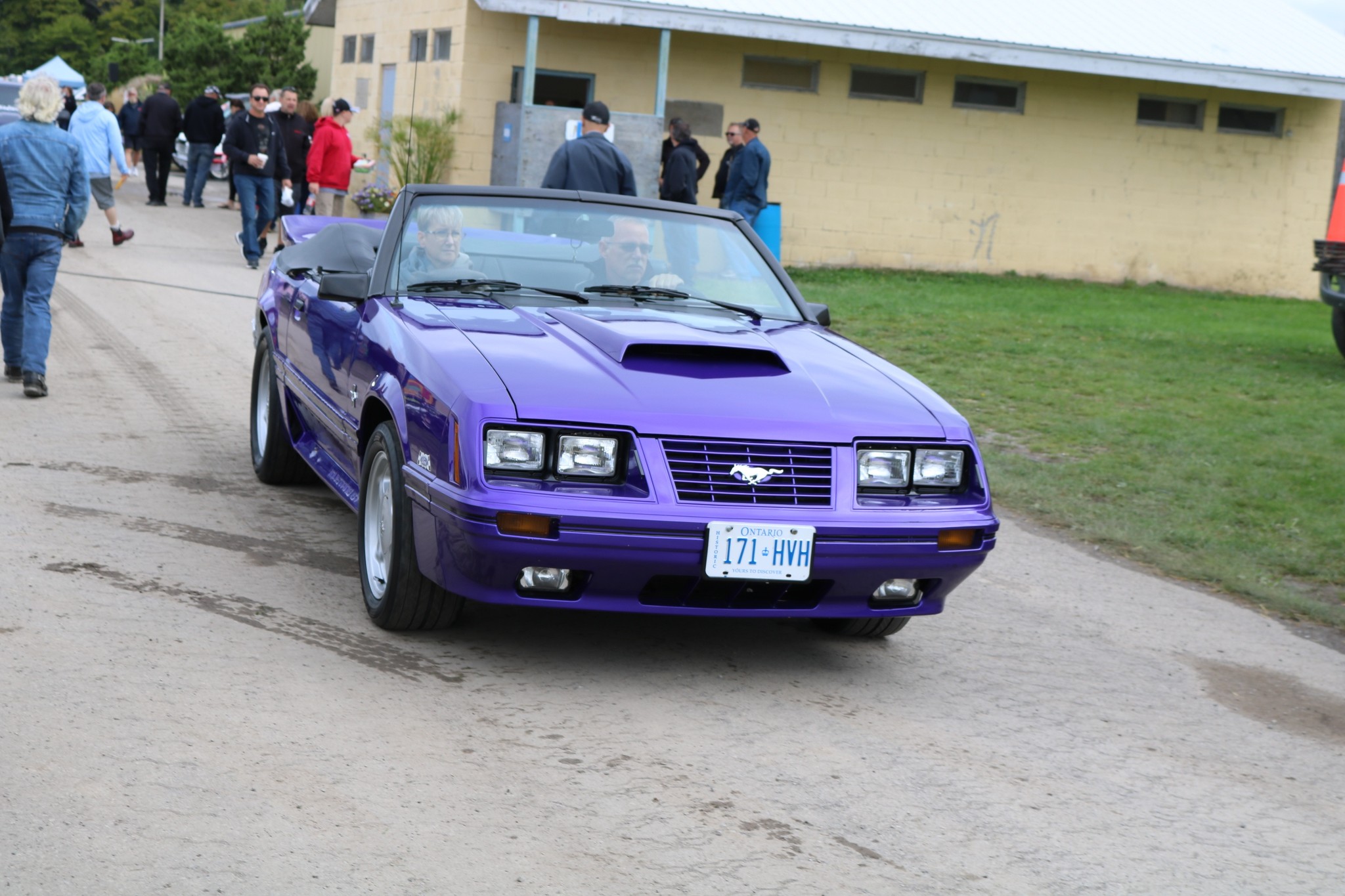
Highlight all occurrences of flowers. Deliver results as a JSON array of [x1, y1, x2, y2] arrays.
[[351, 185, 397, 214]]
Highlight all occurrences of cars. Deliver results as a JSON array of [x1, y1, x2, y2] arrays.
[[173, 90, 252, 179], [0, 73, 27, 125]]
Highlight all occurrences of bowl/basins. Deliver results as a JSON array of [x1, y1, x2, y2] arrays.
[[353, 159, 370, 173]]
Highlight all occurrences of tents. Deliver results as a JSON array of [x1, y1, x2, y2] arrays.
[[22, 54, 84, 89]]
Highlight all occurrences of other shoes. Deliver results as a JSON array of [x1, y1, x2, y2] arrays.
[[245, 260, 259, 269], [22, 370, 48, 398], [259, 237, 267, 257], [194, 203, 204, 208], [69, 239, 84, 247], [273, 244, 285, 254], [3, 365, 23, 383], [235, 232, 248, 260], [111, 228, 134, 245], [146, 199, 167, 206], [182, 200, 190, 206]]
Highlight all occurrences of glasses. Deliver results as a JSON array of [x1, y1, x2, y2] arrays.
[[282, 86, 296, 92], [251, 95, 268, 102], [724, 130, 740, 136], [604, 239, 653, 254], [425, 229, 466, 242]]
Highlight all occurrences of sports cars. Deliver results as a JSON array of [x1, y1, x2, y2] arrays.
[[247, 181, 1002, 642]]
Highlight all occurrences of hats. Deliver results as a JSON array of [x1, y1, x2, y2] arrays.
[[739, 118, 760, 132], [205, 85, 224, 99], [333, 98, 360, 114], [583, 100, 610, 125]]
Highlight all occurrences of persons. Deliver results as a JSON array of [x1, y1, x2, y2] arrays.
[[660, 117, 770, 277], [390, 204, 488, 290], [573, 215, 685, 290], [0, 73, 366, 397], [522, 100, 637, 246]]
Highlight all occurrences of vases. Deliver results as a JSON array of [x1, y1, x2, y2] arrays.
[[359, 212, 395, 220]]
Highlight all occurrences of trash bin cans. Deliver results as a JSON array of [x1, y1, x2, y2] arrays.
[[754, 200, 782, 261]]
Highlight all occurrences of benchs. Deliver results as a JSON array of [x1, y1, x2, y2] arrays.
[[407, 227, 598, 287]]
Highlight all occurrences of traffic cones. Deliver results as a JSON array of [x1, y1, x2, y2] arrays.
[[1310, 158, 1345, 273]]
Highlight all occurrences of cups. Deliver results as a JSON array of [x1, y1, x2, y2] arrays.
[[257, 153, 268, 169]]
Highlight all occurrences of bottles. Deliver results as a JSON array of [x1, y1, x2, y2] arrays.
[[303, 192, 316, 215]]
